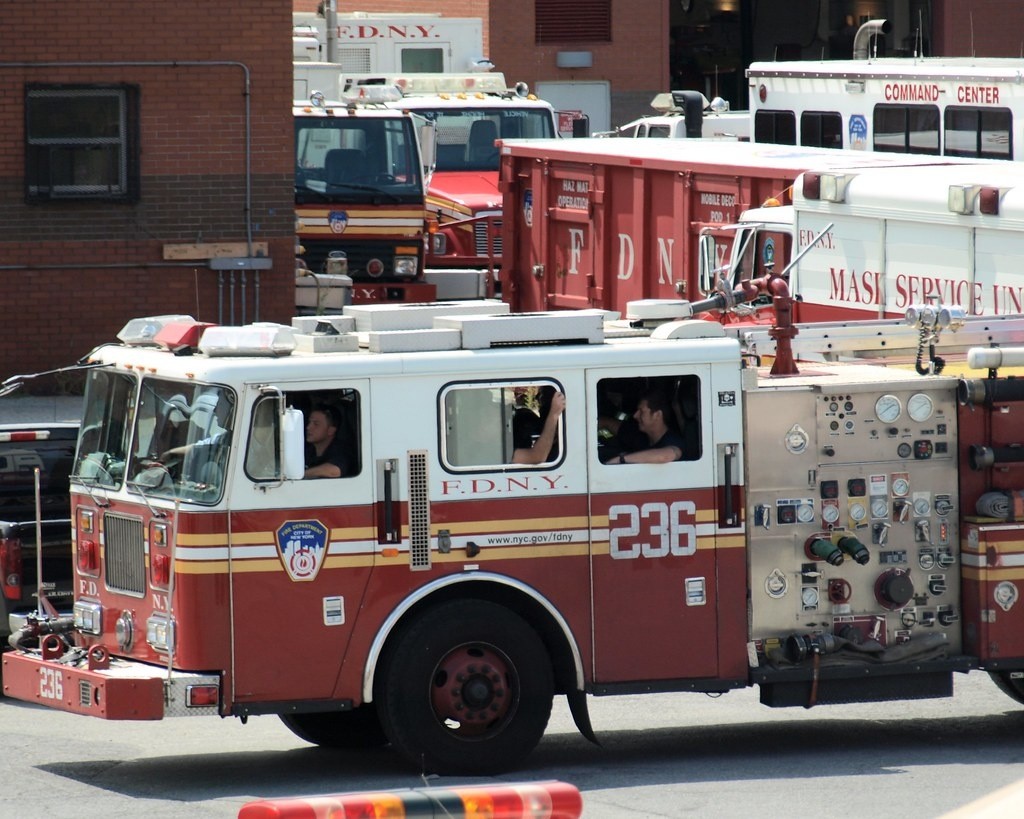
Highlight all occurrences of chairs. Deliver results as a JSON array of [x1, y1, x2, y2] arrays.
[[467, 121, 501, 162], [398, 141, 445, 167], [335, 398, 359, 478], [325, 149, 372, 182]]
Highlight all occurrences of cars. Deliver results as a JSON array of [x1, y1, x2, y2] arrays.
[[0, 367, 93, 610]]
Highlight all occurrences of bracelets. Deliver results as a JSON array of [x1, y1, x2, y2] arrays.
[[619, 452, 625, 463]]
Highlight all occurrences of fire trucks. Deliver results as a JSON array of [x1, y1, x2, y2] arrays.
[[2, 161, 1024, 774], [488, 16, 1023, 337], [291, 0, 595, 307]]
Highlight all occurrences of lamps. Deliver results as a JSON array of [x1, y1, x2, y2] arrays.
[[555, 51, 593, 68]]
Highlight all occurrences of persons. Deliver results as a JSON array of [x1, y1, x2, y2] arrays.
[[304, 406, 346, 478], [512, 387, 566, 465], [161, 389, 233, 488], [597, 394, 687, 465]]
